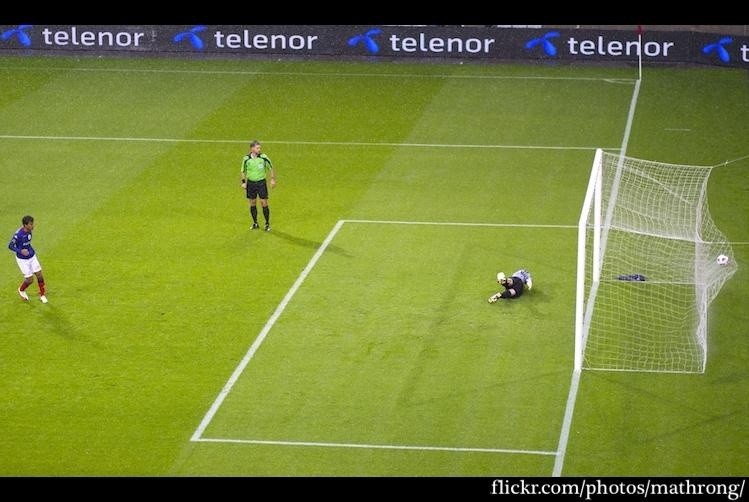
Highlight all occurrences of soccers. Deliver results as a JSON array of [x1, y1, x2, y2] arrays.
[[717, 255, 728, 265]]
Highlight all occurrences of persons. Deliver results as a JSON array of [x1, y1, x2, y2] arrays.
[[487, 268, 533, 304], [241, 140, 276, 231], [8, 215, 49, 304]]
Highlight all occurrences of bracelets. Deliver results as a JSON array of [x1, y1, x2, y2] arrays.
[[242, 179, 245, 184]]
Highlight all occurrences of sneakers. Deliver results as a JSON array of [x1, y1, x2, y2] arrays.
[[18, 289, 29, 301], [250, 223, 270, 231], [40, 295, 48, 303]]
